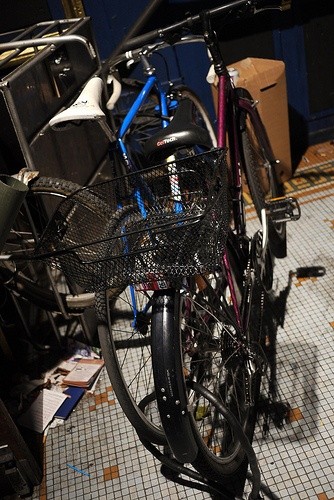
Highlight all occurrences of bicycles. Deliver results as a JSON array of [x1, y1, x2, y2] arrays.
[[0, 1, 326, 493]]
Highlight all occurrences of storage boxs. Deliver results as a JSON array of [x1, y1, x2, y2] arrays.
[[212, 57, 292, 197]]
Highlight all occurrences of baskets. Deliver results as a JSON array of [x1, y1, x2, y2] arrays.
[[12, 145, 231, 292]]
